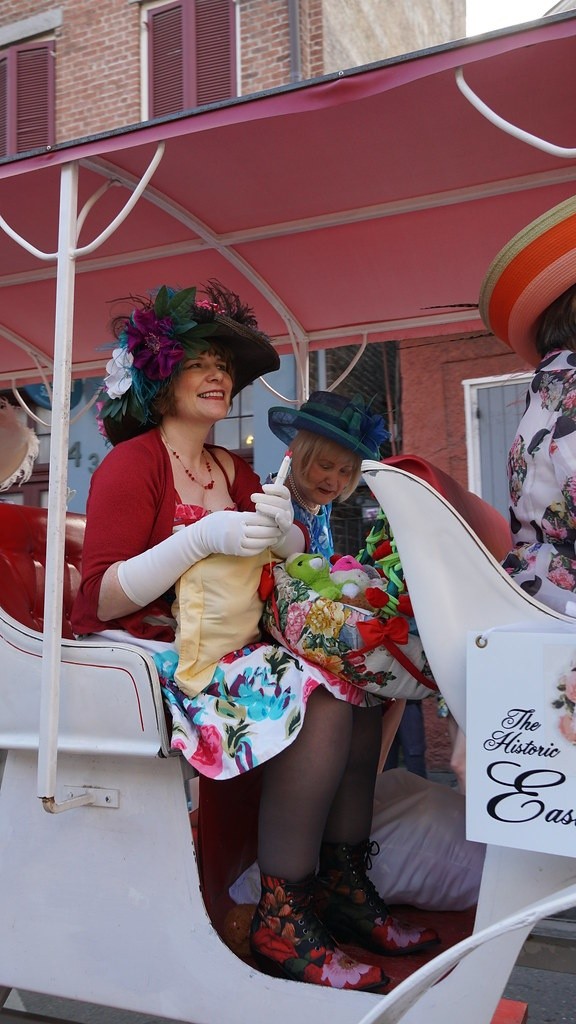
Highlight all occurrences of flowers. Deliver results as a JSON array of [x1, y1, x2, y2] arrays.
[[93, 283, 226, 446]]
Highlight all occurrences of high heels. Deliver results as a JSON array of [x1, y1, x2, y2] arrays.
[[251, 870, 391, 990], [319, 839, 441, 955]]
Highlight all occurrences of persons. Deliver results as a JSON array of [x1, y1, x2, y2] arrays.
[[510, 262, 576, 621], [70, 328, 442, 990], [265, 418, 407, 778]]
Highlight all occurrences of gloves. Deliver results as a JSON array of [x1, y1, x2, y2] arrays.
[[117, 511, 282, 607], [250, 483, 306, 560]]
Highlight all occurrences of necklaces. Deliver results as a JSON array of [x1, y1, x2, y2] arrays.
[[162, 437, 215, 490]]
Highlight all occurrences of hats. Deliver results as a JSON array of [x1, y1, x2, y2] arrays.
[[1, 400, 38, 490], [103, 306, 280, 446], [267, 390, 381, 460], [480, 196, 575, 368]]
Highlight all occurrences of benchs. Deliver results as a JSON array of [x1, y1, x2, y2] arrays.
[[0, 501, 199, 781], [362, 456, 575, 738]]
